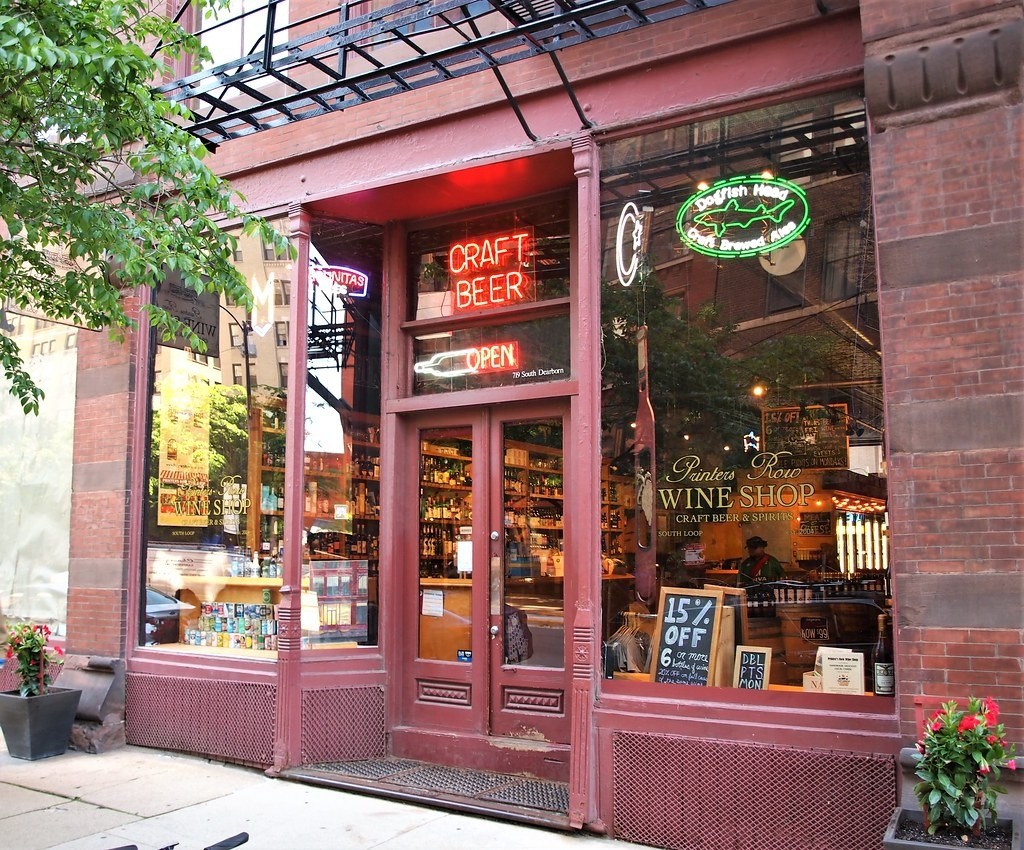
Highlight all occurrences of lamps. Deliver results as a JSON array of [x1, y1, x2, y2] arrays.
[[756, 225, 806, 279]]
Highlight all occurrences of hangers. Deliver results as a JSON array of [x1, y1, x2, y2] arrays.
[[606, 612, 645, 647]]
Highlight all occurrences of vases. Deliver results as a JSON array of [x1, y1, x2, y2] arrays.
[[0, 686, 83, 762], [882, 807, 1021, 850]]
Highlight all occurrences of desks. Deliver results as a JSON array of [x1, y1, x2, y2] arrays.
[[505, 574, 637, 642]]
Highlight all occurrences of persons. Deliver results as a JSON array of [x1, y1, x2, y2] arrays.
[[740, 536, 785, 582]]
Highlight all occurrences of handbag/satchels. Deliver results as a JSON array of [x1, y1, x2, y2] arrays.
[[504, 610, 534, 663]]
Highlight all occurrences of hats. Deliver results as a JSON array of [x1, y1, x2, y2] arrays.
[[745, 536, 767, 549]]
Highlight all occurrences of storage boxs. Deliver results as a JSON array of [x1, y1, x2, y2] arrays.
[[546, 555, 564, 577], [602, 558, 627, 575], [373, 465, 380, 477]]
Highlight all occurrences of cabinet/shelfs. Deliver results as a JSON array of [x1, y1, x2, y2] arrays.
[[245, 389, 639, 580], [178, 574, 358, 661]]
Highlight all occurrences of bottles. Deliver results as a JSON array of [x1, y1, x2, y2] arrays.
[[600, 483, 621, 556], [872, 614, 895, 697], [420, 456, 473, 578], [747, 560, 892, 608], [230, 408, 287, 579], [304, 426, 379, 577], [504, 451, 564, 577]]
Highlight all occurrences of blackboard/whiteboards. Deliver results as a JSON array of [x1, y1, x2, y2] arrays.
[[650, 586, 724, 686], [732, 645, 771, 690], [703, 584, 748, 660]]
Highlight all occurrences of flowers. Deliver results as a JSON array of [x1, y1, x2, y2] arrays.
[[3, 620, 66, 698], [910, 694, 1018, 838]]
